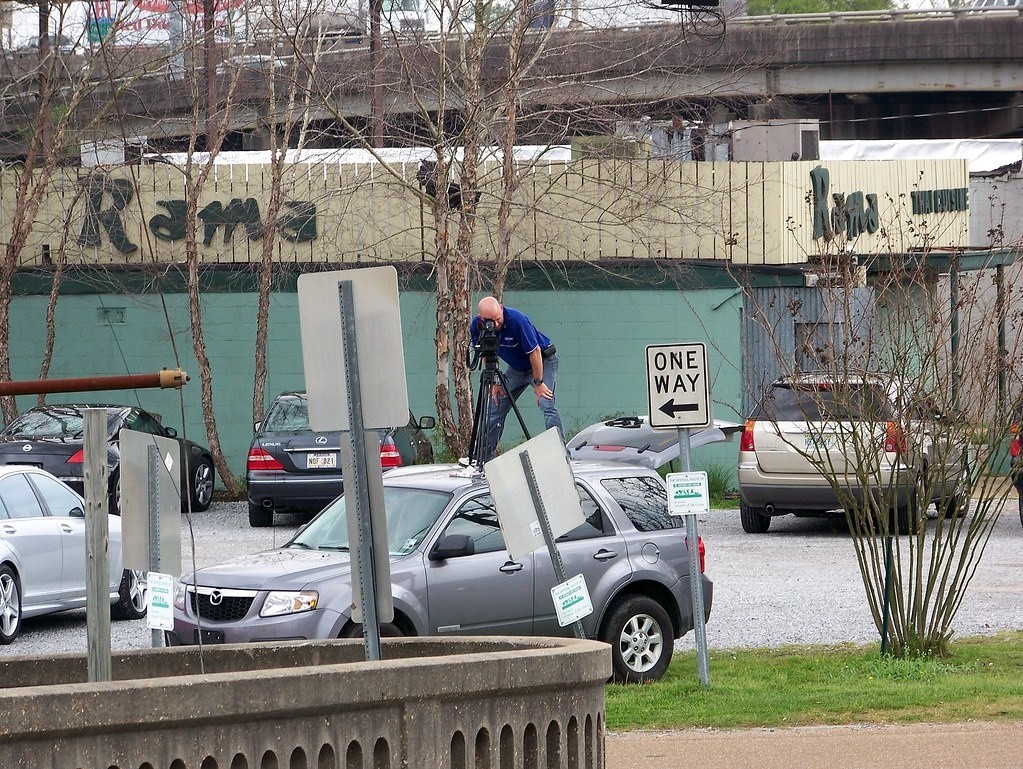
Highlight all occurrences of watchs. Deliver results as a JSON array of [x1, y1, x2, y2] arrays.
[[534, 376, 544, 385]]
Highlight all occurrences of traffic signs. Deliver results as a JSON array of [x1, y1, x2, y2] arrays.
[[645, 341, 713, 432]]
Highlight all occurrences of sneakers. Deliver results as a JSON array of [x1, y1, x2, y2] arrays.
[[458, 456, 484, 466]]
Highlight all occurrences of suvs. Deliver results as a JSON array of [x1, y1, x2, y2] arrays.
[[735, 371, 973, 537], [163, 413, 746, 684], [246, 391, 436, 527]]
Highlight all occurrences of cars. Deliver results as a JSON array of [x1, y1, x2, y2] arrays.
[[1008, 386, 1023, 528], [0, 462, 157, 646], [0, 404, 217, 517]]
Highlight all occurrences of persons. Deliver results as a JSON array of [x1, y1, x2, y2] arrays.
[[456, 296, 572, 462]]
